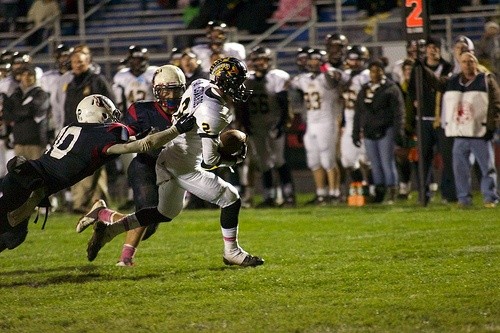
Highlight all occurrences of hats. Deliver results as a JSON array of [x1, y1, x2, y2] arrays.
[[120, 44, 150, 65], [403, 37, 441, 66], [454, 35, 478, 63], [368, 59, 385, 69], [70, 44, 90, 55]]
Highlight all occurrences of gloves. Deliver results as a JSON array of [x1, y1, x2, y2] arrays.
[[395, 134, 408, 147], [221, 143, 248, 167], [175, 113, 196, 134], [352, 132, 360, 147]]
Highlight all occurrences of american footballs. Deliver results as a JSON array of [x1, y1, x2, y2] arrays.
[[218, 129, 246, 152]]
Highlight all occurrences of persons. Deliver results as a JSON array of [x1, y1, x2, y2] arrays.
[[190, 22, 246, 78], [479, 0, 500, 21], [341, 0, 403, 17], [178, 0, 317, 40], [86, 57, 265, 268], [426, 0, 474, 21], [0, 44, 210, 216], [0, 0, 100, 52], [0, 94, 195, 254], [74, 64, 188, 266], [237, 19, 500, 209]]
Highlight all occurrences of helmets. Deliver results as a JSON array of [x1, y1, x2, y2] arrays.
[[170, 48, 182, 58], [152, 65, 187, 103], [75, 94, 122, 124], [206, 20, 230, 45], [56, 44, 70, 56], [210, 57, 254, 104], [295, 33, 371, 60], [0, 51, 30, 72], [250, 46, 273, 72]]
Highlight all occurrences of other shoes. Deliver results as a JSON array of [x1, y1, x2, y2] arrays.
[[255, 197, 296, 208], [222, 248, 264, 267], [374, 190, 384, 203], [115, 260, 137, 267], [76, 199, 107, 233], [304, 194, 343, 207], [86, 220, 109, 261], [484, 200, 495, 208]]
